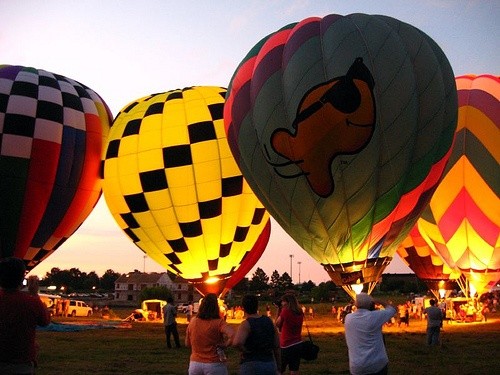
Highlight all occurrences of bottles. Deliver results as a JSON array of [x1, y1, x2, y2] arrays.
[[217, 348, 227, 361]]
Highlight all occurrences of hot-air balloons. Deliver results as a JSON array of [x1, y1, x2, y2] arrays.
[[100, 85, 271, 317], [223, 13, 459, 304], [395, 223, 459, 318], [415, 73, 499, 323], [183, 219, 271, 309], [0, 65, 114, 290]]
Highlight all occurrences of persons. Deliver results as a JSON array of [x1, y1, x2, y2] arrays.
[[0, 257, 53, 375], [185, 293, 233, 375], [229, 295, 285, 375], [297, 303, 345, 321], [52, 296, 70, 318], [419, 298, 444, 349], [92, 304, 114, 320], [271, 292, 305, 375], [162, 298, 184, 350], [344, 294, 397, 375], [129, 300, 272, 318], [373, 282, 500, 323]]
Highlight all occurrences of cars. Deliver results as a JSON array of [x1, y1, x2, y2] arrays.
[[177, 304, 190, 313], [339, 305, 352, 323]]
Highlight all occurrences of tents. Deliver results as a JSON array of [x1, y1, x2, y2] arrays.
[[141, 299, 167, 323]]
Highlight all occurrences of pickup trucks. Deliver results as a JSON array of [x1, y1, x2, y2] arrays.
[[68, 300, 93, 318]]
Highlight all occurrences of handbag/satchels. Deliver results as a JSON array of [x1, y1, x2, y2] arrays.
[[301, 341, 319, 361]]
